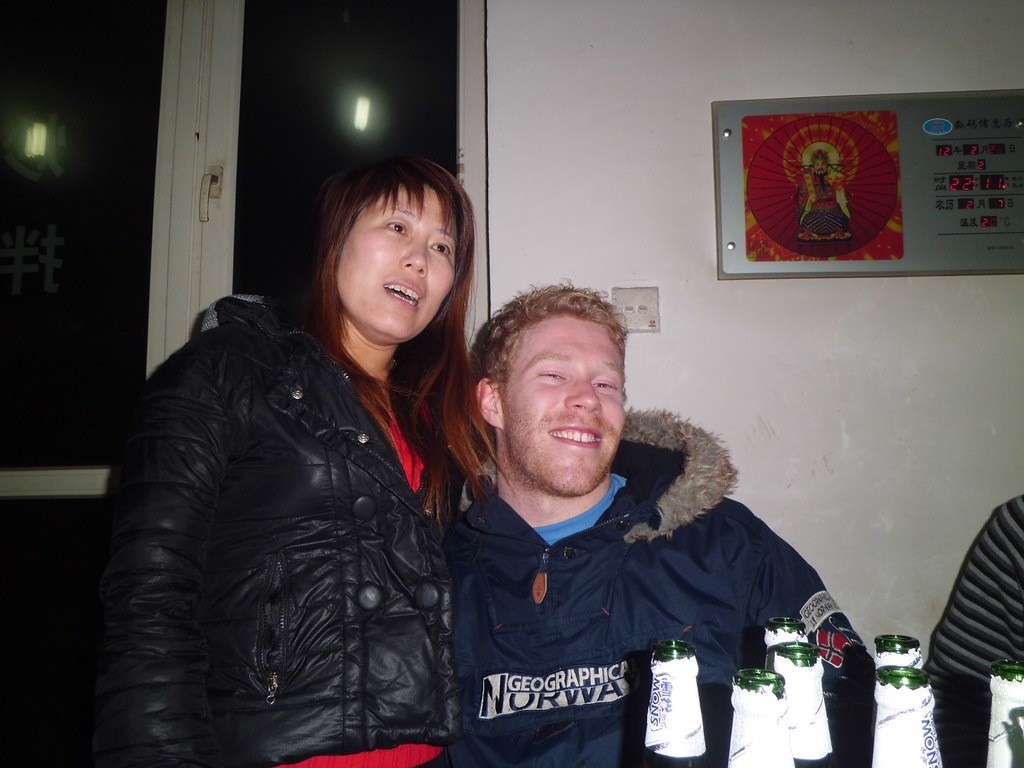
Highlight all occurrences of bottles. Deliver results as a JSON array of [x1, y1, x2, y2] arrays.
[[639, 616, 1024, 768]]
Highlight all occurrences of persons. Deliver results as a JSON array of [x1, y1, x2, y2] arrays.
[[94, 155, 503, 768], [923, 493, 1024, 768], [439, 287, 876, 768]]
